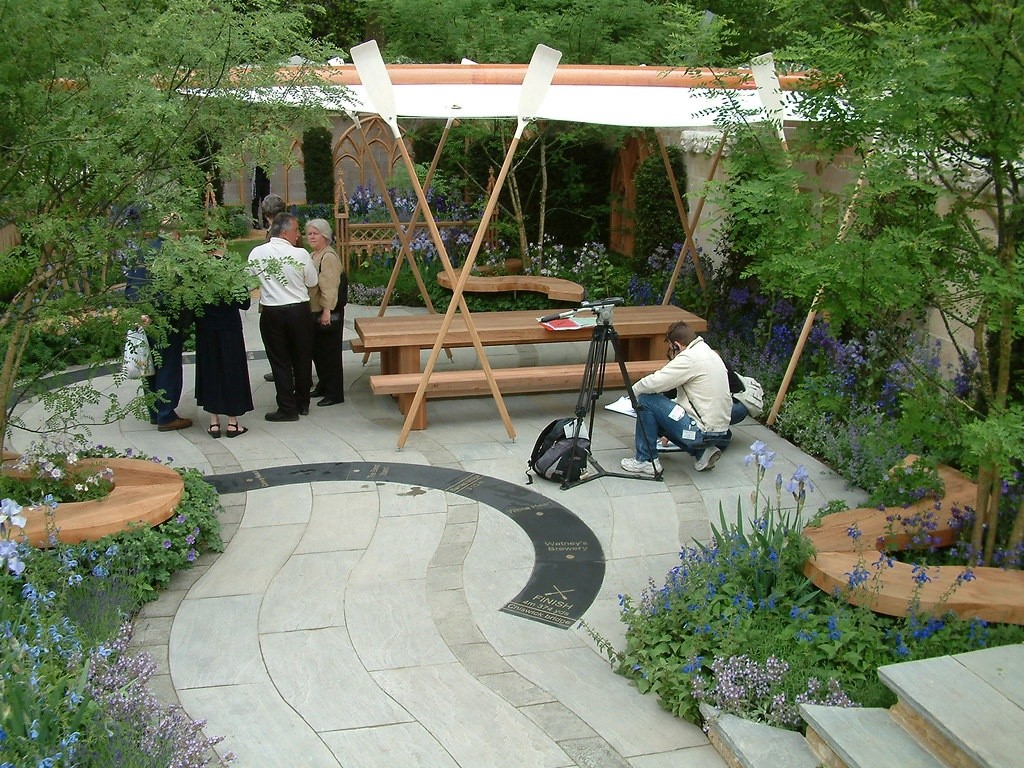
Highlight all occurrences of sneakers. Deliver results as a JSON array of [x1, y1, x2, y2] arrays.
[[693, 446, 722, 472], [621, 457, 664, 475]]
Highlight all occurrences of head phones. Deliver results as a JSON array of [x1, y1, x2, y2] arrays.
[[667, 334, 680, 350]]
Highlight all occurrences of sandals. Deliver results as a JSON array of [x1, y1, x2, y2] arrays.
[[207, 423, 221, 438], [226, 422, 248, 438]]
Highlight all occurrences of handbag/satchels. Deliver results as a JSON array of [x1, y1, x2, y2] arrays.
[[123, 325, 156, 379], [732, 377, 764, 418], [318, 251, 348, 313]]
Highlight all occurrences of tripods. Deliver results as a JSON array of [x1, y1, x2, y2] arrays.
[[541, 305, 664, 482]]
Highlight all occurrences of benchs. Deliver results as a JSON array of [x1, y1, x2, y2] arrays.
[[370, 358, 687, 431], [437, 259, 584, 302], [350, 338, 381, 366], [0, 452, 185, 550], [799, 453, 1024, 625]]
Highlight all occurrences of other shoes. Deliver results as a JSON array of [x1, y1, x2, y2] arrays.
[[293, 376, 313, 390], [317, 397, 344, 406], [150, 418, 158, 424], [310, 389, 322, 397], [265, 410, 299, 421], [300, 408, 309, 415], [264, 373, 275, 381], [158, 417, 193, 431]]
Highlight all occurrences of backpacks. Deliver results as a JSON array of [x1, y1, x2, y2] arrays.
[[525, 418, 592, 485]]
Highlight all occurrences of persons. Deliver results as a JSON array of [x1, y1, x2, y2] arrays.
[[656, 344, 765, 447], [258, 194, 313, 383], [244, 212, 319, 422], [125, 212, 255, 439], [621, 319, 733, 475], [305, 218, 349, 406]]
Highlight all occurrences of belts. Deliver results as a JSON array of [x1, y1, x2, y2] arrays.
[[264, 301, 310, 310]]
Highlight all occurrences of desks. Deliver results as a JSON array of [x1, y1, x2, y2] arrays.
[[354, 305, 707, 414]]
